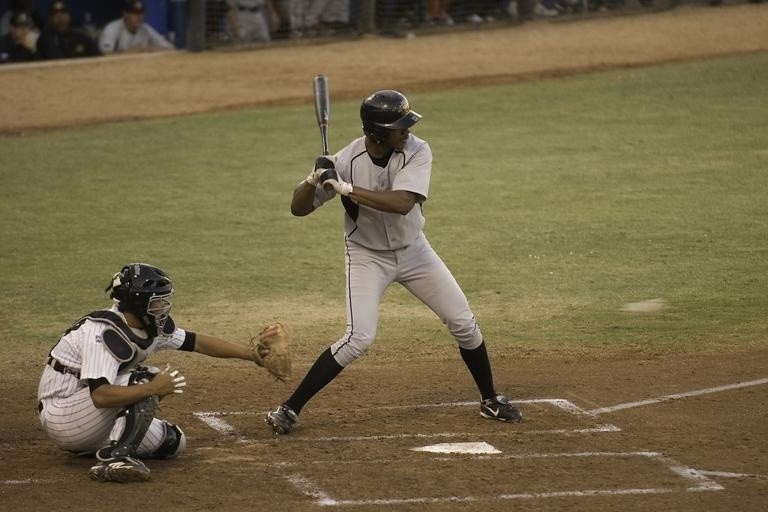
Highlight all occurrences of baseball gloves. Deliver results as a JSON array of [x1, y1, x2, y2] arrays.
[[256, 325, 292, 377]]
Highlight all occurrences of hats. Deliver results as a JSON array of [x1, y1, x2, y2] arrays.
[[9, 0, 144, 28]]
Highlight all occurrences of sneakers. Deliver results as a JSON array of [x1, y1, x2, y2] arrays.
[[88, 458, 153, 484], [480, 395, 522, 422], [263, 402, 298, 434]]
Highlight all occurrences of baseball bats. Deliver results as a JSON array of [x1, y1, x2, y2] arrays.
[[312, 76, 335, 191]]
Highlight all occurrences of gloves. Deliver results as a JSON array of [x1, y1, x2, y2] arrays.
[[304, 154, 354, 198]]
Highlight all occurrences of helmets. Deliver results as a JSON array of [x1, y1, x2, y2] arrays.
[[103, 262, 177, 338], [357, 88, 423, 131]]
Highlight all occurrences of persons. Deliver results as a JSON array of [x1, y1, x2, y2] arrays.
[[36, 262, 293, 484], [265, 89, 524, 436], [213, 0, 587, 42], [1, 0, 173, 65]]
